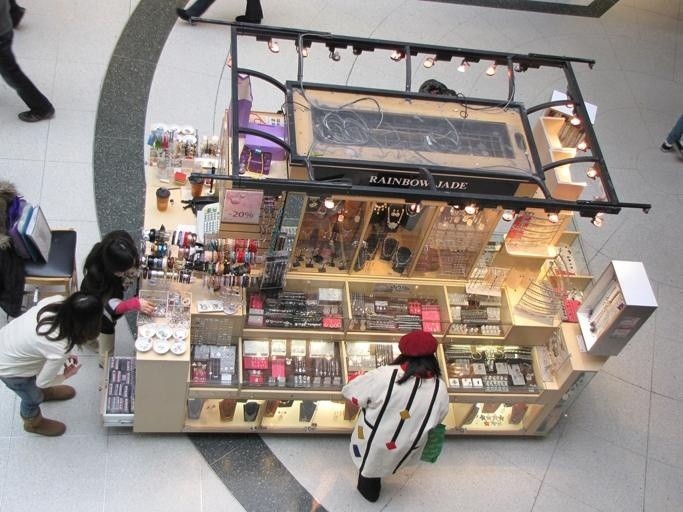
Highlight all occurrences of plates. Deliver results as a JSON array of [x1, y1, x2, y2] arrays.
[[134, 323, 188, 355]]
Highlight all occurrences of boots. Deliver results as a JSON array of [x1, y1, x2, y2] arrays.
[[21, 408, 65, 436], [40, 385, 75, 401]]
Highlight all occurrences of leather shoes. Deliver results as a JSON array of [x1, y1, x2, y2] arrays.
[[19, 109, 55, 121]]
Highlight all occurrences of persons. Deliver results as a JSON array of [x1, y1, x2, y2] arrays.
[[340, 331, 449, 503], [175, 0, 263, 24], [0, 1, 55, 123], [660, 112, 683, 165], [0, 291, 101, 436], [79, 229, 156, 371]]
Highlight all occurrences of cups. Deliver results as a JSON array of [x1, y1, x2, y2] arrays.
[[155, 188, 170, 212], [189, 175, 204, 197]]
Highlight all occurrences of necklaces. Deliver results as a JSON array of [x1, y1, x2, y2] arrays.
[[190, 289, 344, 389], [345, 291, 539, 388], [293, 197, 490, 281]]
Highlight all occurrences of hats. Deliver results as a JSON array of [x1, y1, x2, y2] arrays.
[[398, 330, 437, 357]]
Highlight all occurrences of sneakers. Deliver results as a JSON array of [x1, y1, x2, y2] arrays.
[[661, 142, 674, 152], [86, 338, 99, 353], [236, 15, 261, 23], [175, 7, 196, 22]]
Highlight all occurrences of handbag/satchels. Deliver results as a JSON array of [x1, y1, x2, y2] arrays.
[[8, 196, 31, 258], [421, 424, 445, 462]]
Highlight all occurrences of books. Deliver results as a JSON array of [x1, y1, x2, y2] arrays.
[[10, 203, 52, 262]]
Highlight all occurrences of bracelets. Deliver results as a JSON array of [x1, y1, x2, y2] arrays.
[[141, 225, 266, 290]]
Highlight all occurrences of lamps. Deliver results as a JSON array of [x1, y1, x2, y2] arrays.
[[566, 83, 583, 128], [253, 36, 528, 75]]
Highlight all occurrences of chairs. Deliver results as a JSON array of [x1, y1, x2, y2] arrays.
[[16, 230, 77, 298]]
